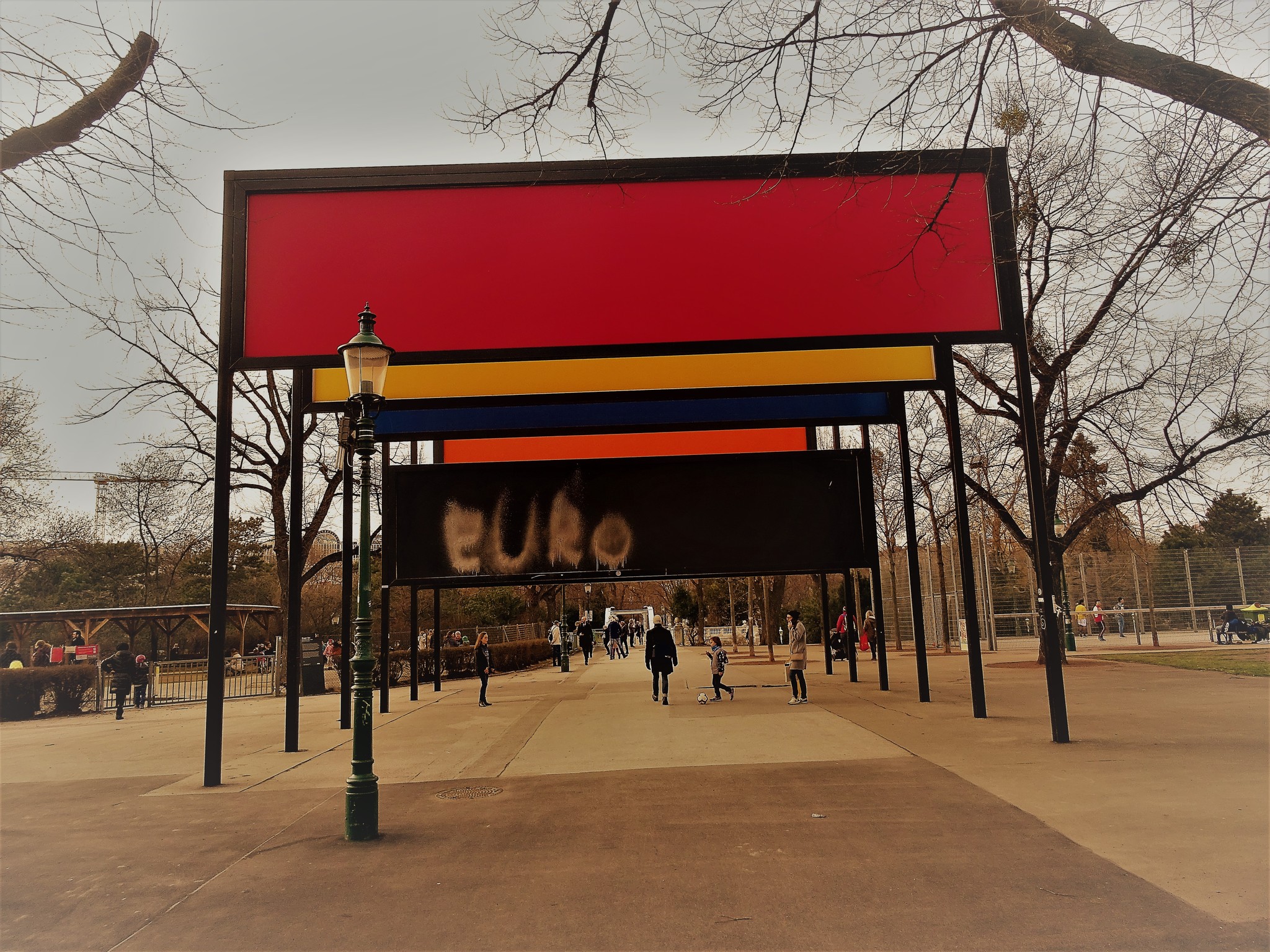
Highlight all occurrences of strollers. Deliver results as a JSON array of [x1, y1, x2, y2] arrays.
[[830, 628, 858, 662]]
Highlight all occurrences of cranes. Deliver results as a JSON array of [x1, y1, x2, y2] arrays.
[[0, 470, 173, 545]]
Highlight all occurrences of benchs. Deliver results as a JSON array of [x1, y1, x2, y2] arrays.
[[1218, 631, 1257, 645]]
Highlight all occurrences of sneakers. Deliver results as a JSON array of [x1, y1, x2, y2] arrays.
[[799, 696, 808, 703], [788, 695, 800, 705]]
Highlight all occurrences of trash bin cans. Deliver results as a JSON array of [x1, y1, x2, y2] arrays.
[[1240, 602, 1269, 641]]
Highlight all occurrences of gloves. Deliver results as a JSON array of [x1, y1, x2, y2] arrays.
[[673, 661, 678, 667], [646, 663, 651, 670]]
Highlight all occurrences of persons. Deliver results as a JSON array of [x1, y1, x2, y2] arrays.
[[576, 617, 594, 665], [837, 607, 857, 662], [474, 632, 495, 707], [101, 642, 149, 720], [1074, 599, 1088, 638], [863, 610, 877, 661], [1215, 604, 1238, 645], [170, 643, 179, 661], [1113, 597, 1127, 638], [1092, 600, 1108, 641], [322, 639, 341, 670], [225, 641, 273, 676], [547, 620, 562, 667], [645, 615, 678, 705], [0, 630, 85, 669], [706, 636, 735, 701], [786, 610, 808, 705], [602, 616, 646, 660], [417, 628, 470, 650]]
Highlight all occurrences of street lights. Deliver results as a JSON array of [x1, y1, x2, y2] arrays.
[[583, 583, 592, 625], [661, 604, 665, 626], [336, 300, 397, 842]]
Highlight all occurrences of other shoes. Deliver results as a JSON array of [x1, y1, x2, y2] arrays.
[[134, 704, 140, 708], [482, 697, 492, 706], [622, 652, 628, 658], [478, 698, 486, 707], [140, 704, 144, 709], [617, 653, 621, 659], [728, 688, 735, 700], [652, 694, 658, 701], [662, 696, 669, 705], [710, 696, 722, 701], [613, 655, 615, 659], [235, 672, 240, 676], [610, 655, 613, 660]]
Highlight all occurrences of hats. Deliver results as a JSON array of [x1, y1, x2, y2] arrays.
[[455, 631, 461, 635], [136, 655, 146, 663], [553, 620, 561, 624], [328, 639, 333, 643], [653, 615, 662, 624]]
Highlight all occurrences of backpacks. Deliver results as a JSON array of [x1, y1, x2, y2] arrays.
[[548, 626, 557, 644]]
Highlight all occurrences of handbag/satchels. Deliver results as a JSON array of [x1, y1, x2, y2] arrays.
[[859, 629, 869, 652], [784, 660, 792, 682]]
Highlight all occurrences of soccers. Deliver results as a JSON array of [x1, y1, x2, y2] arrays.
[[697, 692, 708, 705]]
